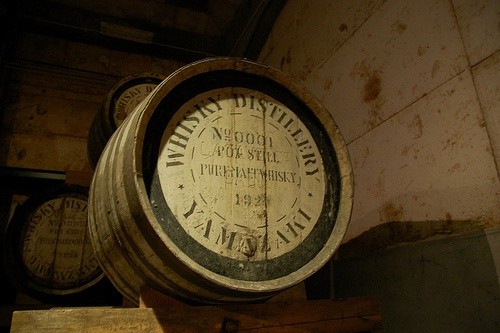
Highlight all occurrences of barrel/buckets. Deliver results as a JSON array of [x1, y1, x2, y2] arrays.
[[87, 72, 168, 169], [4, 184, 108, 297], [88, 58, 356, 308]]
